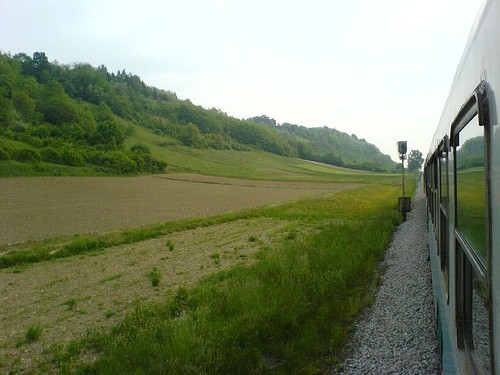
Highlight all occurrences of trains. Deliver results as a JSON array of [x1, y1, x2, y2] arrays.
[[423, 0, 499, 374]]
